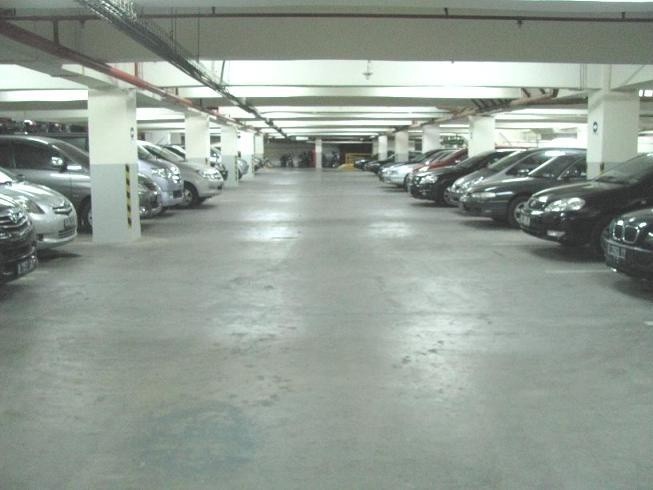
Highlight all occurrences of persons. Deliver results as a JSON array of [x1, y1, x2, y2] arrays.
[[279, 149, 345, 168]]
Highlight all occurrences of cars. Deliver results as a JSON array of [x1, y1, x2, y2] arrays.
[[408, 150, 520, 203], [354, 150, 395, 172], [520, 152, 652, 260], [136, 141, 224, 207], [1, 193, 37, 280], [455, 150, 587, 229], [601, 208, 653, 279], [165, 142, 264, 182], [0, 166, 79, 249], [448, 149, 583, 205], [363, 151, 422, 174], [376, 149, 469, 192]]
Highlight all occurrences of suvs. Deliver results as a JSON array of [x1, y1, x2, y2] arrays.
[[18, 131, 185, 217], [2, 132, 164, 234]]
